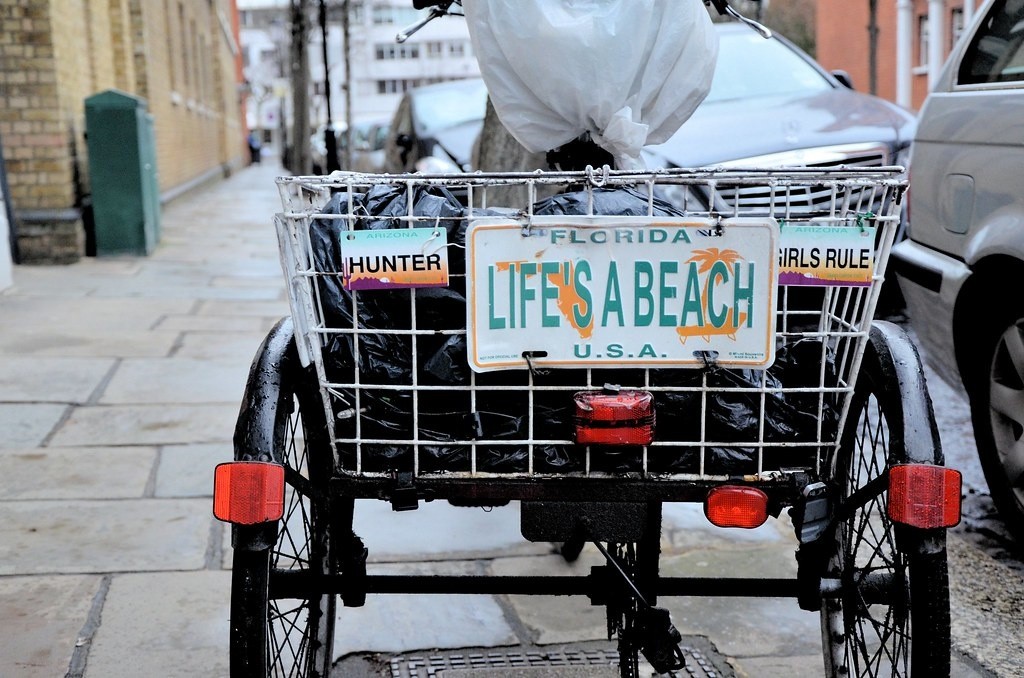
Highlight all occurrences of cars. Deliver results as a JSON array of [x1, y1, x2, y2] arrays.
[[384, 78, 489, 208], [887, 1, 1024, 551], [309, 117, 394, 193], [638, 20, 918, 286]]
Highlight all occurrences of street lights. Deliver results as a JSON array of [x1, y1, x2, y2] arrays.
[[269, 18, 288, 147]]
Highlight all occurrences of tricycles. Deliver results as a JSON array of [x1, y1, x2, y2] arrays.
[[212, 1, 961, 678]]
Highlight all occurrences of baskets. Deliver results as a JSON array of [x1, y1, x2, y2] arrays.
[[273, 164, 912, 480]]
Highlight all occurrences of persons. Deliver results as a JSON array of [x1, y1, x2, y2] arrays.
[[310, 128, 328, 176], [246, 130, 261, 162]]
